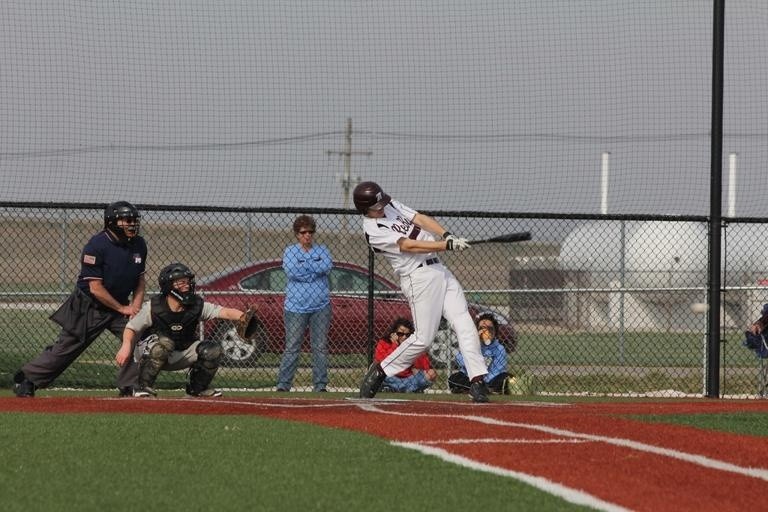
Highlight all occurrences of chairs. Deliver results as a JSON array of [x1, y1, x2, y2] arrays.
[[744, 303, 767, 398]]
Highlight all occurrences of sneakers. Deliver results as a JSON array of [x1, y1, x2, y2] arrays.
[[13, 382, 31, 397], [199, 390, 221, 397], [118, 386, 156, 397], [470, 381, 487, 402], [360, 362, 386, 398]]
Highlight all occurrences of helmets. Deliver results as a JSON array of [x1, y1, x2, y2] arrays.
[[160, 263, 196, 306], [353, 181, 390, 210], [103, 201, 140, 244]]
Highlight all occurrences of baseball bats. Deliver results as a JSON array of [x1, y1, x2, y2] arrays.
[[463, 231, 532, 244]]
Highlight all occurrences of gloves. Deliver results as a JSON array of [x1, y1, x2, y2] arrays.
[[443, 232, 470, 252]]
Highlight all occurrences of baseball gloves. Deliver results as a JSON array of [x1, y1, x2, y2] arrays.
[[234, 305, 260, 340]]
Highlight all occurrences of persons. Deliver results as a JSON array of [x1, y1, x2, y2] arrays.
[[352, 181, 491, 403], [12, 200, 148, 398], [447, 312, 527, 396], [114, 262, 251, 398], [740, 302, 768, 359], [366, 317, 438, 394], [274, 214, 333, 394]]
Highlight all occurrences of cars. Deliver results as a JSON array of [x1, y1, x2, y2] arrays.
[[193, 258, 518, 367]]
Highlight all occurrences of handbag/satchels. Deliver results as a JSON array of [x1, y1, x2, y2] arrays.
[[509, 369, 535, 395]]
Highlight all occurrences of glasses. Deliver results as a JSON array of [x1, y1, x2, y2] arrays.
[[396, 332, 410, 337], [298, 230, 313, 234]]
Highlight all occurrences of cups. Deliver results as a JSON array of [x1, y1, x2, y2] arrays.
[[482, 329, 492, 346]]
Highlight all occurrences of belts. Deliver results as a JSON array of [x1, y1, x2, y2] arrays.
[[418, 257, 438, 266]]
